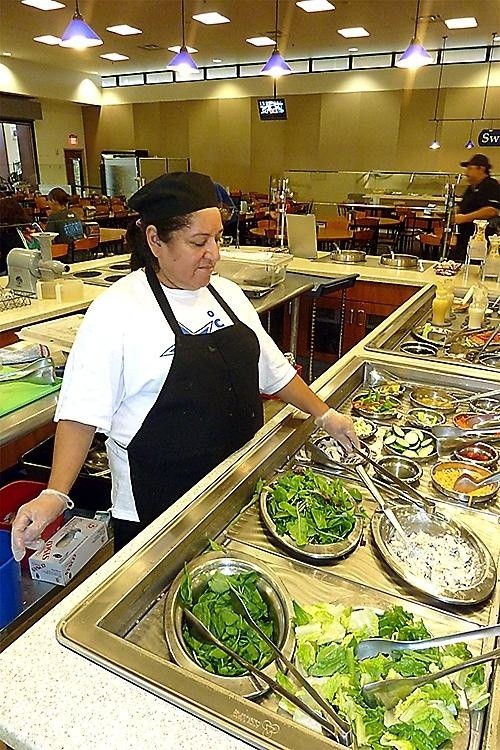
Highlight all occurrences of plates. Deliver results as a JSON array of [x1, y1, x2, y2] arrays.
[[429, 303, 493, 331]]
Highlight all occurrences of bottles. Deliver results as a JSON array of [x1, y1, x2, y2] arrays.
[[484, 234, 500, 279], [240, 200, 248, 214], [468, 287, 489, 329], [467, 219, 488, 259], [434, 279, 455, 325]]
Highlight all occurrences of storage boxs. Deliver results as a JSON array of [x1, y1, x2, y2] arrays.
[[214, 244, 294, 287], [18, 425, 111, 510]]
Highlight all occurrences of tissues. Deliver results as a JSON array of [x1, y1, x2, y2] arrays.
[[28, 516, 108, 587]]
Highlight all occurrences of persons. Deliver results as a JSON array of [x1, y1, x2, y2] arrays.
[[213, 182, 241, 241], [10, 169, 362, 563], [40, 188, 87, 265], [450, 154, 499, 265]]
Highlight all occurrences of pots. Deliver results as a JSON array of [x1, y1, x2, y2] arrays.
[[380, 253, 420, 271], [330, 248, 367, 264]]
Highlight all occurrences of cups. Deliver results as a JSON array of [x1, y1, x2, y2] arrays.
[[35, 277, 64, 301], [56, 279, 85, 303]]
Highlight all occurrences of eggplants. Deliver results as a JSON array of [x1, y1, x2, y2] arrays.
[[383, 424, 435, 459]]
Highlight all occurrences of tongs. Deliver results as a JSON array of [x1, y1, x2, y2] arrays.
[[359, 623, 500, 710], [179, 585, 354, 748]]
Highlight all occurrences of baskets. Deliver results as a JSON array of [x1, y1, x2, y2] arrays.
[[1, 288, 36, 311]]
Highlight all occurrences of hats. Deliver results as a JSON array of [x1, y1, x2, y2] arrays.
[[127, 171, 220, 228], [459, 154, 488, 167]]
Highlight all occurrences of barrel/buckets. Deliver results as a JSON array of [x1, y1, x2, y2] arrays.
[[0, 530, 22, 630]]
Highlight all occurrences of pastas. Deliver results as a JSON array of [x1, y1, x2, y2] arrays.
[[435, 467, 494, 506]]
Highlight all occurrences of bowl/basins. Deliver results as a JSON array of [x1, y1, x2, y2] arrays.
[[161, 547, 299, 700], [367, 506, 499, 609], [309, 383, 500, 505], [400, 324, 499, 367], [257, 473, 367, 563], [83, 438, 111, 478]]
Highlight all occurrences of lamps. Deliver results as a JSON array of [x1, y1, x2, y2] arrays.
[[58, 0, 110, 49], [168, 0, 206, 79], [392, 0, 436, 75], [68, 132, 79, 146], [258, 0, 296, 80], [427, 30, 499, 158]]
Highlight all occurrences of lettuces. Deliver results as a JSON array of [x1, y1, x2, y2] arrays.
[[292, 600, 490, 749]]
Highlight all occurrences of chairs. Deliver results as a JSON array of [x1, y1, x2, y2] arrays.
[[0, 167, 500, 278]]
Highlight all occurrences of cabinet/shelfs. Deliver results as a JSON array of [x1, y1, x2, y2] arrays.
[[283, 280, 420, 367]]
